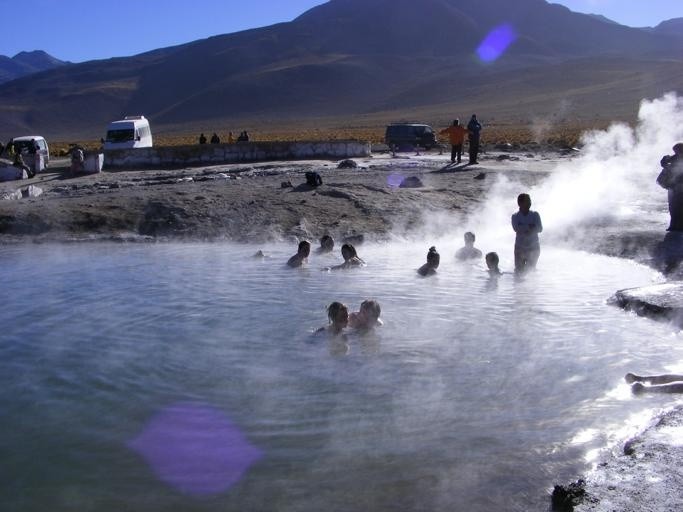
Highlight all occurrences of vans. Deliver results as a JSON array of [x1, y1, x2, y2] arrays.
[[385, 122, 436, 150], [5, 135, 50, 167], [100, 115, 153, 150]]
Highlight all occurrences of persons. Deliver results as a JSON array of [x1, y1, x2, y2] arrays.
[[321, 243, 371, 274], [346, 298, 386, 329], [314, 235, 337, 257], [5, 138, 16, 162], [482, 250, 507, 274], [26, 139, 36, 154], [465, 113, 481, 165], [227, 130, 248, 145], [434, 119, 476, 163], [198, 133, 207, 145], [657, 141, 682, 232], [326, 335, 351, 360], [12, 148, 35, 179], [284, 239, 311, 272], [477, 275, 502, 293], [359, 332, 383, 356], [414, 245, 442, 279], [510, 193, 544, 275], [452, 231, 483, 262], [309, 299, 351, 341], [209, 132, 220, 144]]
